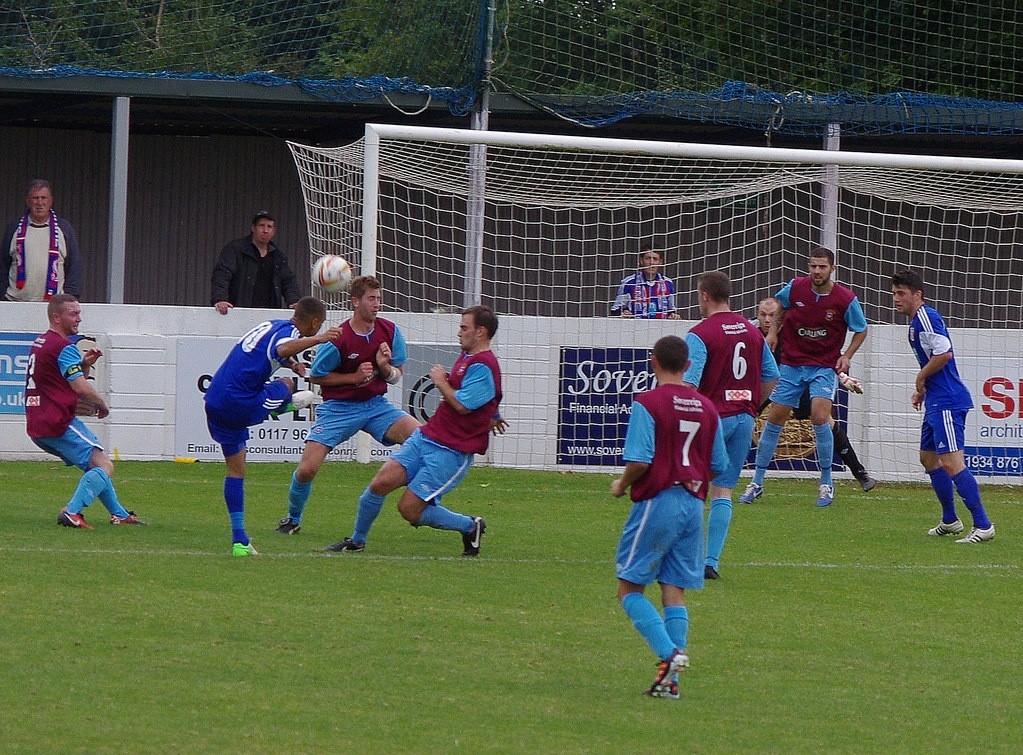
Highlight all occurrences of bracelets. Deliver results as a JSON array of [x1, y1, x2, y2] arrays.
[[493, 413, 500, 419]]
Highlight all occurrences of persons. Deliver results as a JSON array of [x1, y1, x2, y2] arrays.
[[683, 273, 779, 580], [0, 179, 84, 303], [610, 243, 682, 319], [611, 335, 729, 700], [210, 211, 302, 315], [893, 269, 997, 545], [25, 295, 149, 529], [754, 297, 876, 493], [276, 275, 441, 537], [204, 296, 342, 557], [739, 248, 868, 507], [313, 305, 509, 556]]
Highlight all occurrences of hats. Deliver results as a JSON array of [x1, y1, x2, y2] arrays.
[[252, 210, 276, 223]]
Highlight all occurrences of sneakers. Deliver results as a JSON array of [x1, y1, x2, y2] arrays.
[[644, 682, 678, 699], [649, 649, 690, 697], [739, 483, 763, 503], [232, 542, 258, 557], [274, 518, 300, 535], [110, 510, 149, 525], [954, 523, 996, 542], [928, 517, 964, 536], [817, 479, 835, 507], [57, 508, 94, 530], [462, 515, 485, 555], [321, 537, 365, 553], [852, 465, 876, 491], [272, 391, 314, 415]]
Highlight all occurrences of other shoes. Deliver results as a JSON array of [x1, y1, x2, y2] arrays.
[[704, 565, 718, 579]]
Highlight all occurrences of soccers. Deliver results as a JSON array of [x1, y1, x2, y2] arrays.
[[312, 254, 351, 293]]
